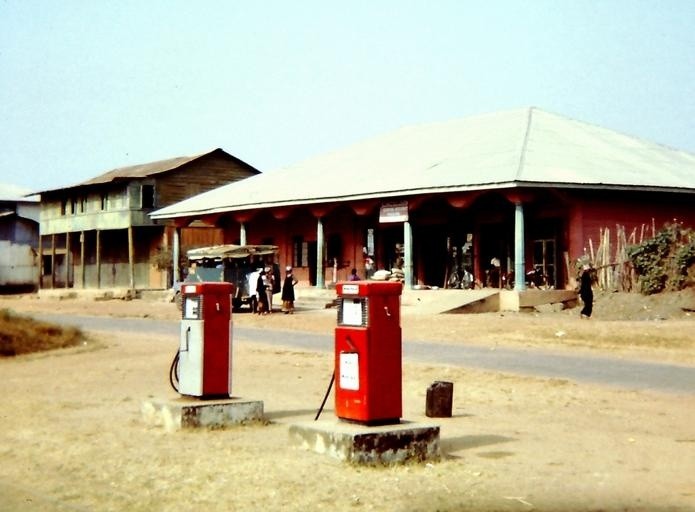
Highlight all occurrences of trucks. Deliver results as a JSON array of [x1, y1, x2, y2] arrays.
[[173, 244, 282, 315]]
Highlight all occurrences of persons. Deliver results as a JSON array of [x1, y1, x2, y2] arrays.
[[580, 264, 597, 319], [281, 266, 298, 314], [248, 267, 275, 315], [349, 269, 361, 281]]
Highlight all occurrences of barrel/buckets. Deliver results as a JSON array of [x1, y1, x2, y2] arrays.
[[426, 381, 454, 418]]
